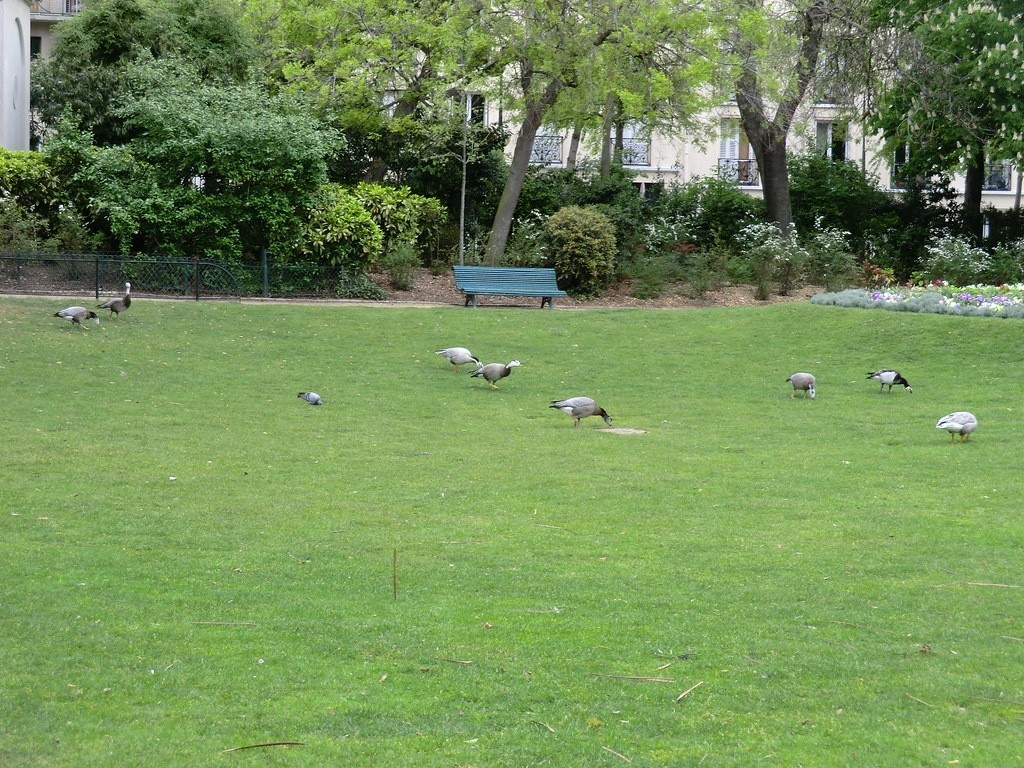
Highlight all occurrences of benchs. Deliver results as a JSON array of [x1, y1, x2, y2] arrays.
[[451, 265, 567, 311]]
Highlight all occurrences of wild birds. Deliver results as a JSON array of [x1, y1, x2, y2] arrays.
[[433, 347, 524, 390], [864, 369, 913, 393], [53, 283, 132, 329], [549, 396, 612, 427], [935, 411, 977, 442], [785, 371, 815, 399], [297, 392, 323, 406]]
